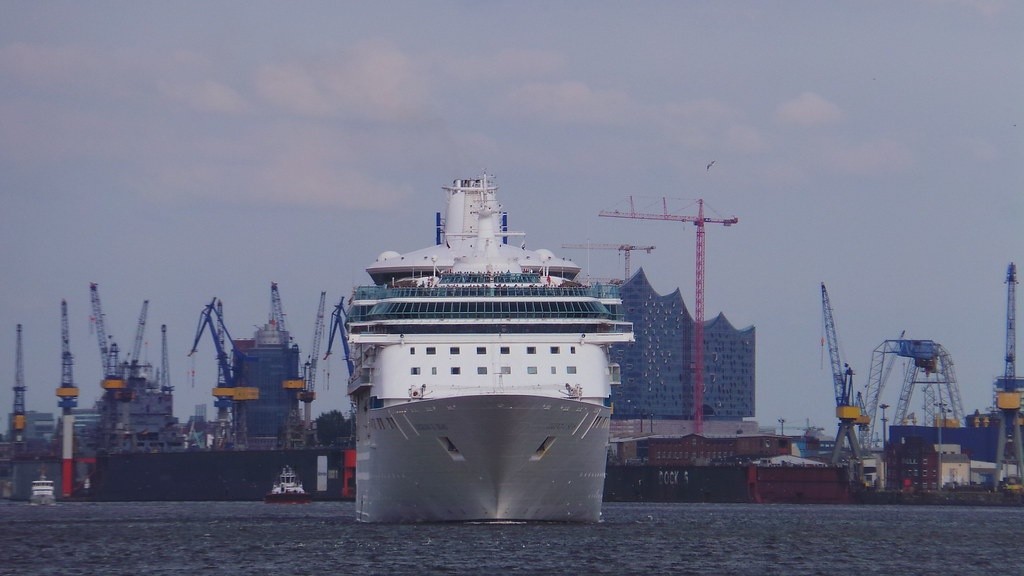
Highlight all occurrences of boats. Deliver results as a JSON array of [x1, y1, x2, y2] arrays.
[[743, 457, 853, 507]]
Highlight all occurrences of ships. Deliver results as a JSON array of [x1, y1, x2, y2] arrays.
[[343, 170, 638, 527]]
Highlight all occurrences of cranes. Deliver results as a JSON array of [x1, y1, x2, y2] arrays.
[[9, 281, 331, 504], [560, 238, 655, 281], [601, 193, 739, 435], [820, 261, 1024, 497]]
